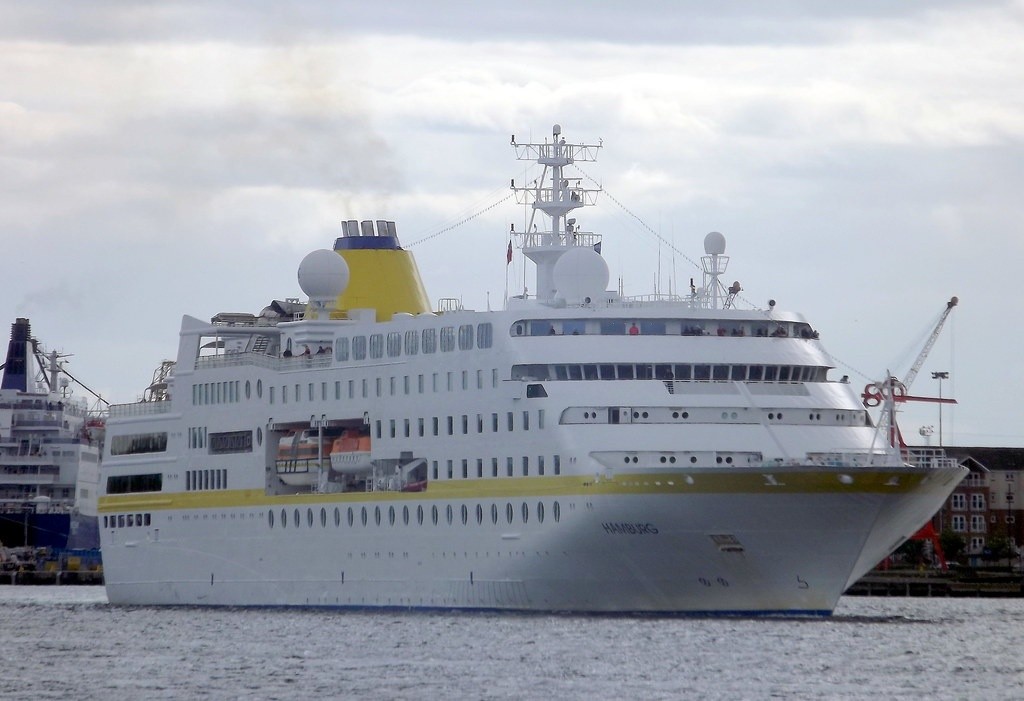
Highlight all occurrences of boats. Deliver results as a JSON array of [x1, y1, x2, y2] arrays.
[[275, 428, 334, 488], [329, 430, 372, 476], [1, 319, 110, 587]]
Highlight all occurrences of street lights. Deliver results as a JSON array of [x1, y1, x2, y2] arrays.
[[932, 371, 949, 448]]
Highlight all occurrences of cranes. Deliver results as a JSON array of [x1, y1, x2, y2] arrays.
[[864, 296, 959, 444]]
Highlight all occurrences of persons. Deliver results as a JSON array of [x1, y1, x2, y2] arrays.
[[549, 325, 556, 335], [666, 369, 674, 379], [283, 349, 292, 357], [299, 346, 311, 367], [684, 324, 744, 337], [757, 327, 786, 337], [840, 375, 848, 381], [630, 322, 639, 334], [573, 328, 580, 335], [801, 327, 819, 339], [316, 346, 325, 366]]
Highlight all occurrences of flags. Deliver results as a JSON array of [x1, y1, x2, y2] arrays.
[[594, 242, 601, 254], [507, 243, 512, 263]]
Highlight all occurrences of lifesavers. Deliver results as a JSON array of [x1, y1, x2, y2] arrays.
[[864, 384, 881, 395], [864, 397, 880, 407]]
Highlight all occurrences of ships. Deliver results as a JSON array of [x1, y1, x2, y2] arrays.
[[98, 124, 971, 624]]
